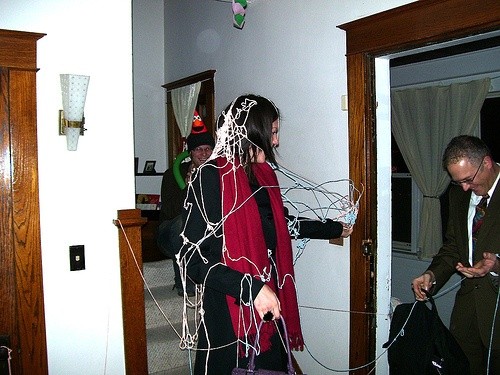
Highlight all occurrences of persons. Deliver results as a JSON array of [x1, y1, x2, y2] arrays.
[[156, 130, 214, 297], [174, 94, 354, 375], [411, 135, 500, 375]]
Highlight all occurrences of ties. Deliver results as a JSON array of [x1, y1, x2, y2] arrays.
[[472, 194, 490, 267]]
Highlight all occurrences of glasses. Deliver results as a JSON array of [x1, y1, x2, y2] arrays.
[[450, 155, 485, 185], [193, 147, 213, 153]]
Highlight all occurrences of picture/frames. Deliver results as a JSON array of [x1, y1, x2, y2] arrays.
[[142, 161, 157, 174]]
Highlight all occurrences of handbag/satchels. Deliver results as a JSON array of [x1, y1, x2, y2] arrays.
[[233, 314, 294, 375]]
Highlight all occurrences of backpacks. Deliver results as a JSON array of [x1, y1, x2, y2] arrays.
[[387, 288, 470, 375]]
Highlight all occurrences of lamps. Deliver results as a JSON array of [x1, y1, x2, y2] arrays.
[[59, 72, 90, 152]]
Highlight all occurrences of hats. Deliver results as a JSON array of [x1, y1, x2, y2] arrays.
[[187, 131, 215, 152]]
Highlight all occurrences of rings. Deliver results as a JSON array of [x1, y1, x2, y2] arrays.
[[263, 311, 274, 321]]
